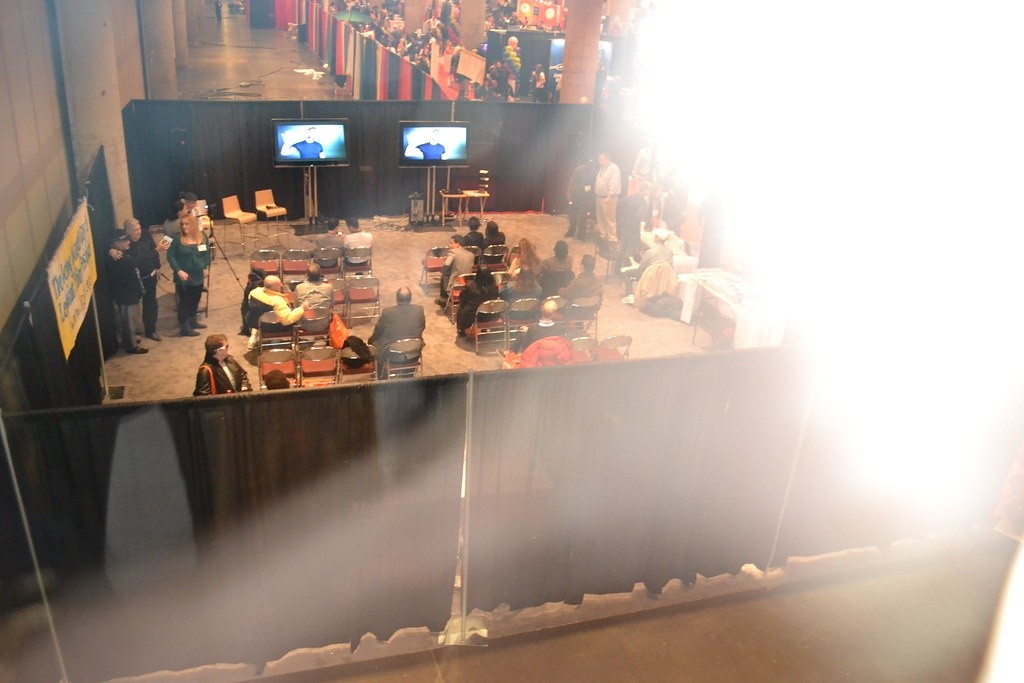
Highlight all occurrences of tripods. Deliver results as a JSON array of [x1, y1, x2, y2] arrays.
[[207, 214, 245, 317]]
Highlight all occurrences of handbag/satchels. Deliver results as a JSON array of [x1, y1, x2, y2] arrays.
[[328, 314, 349, 348]]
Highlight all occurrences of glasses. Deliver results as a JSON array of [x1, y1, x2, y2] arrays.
[[213, 344, 228, 352]]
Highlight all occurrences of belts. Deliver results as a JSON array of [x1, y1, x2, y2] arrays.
[[595, 194, 608, 198]]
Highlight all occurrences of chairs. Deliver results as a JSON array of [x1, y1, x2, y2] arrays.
[[420, 243, 630, 372], [222, 194, 257, 247], [248, 243, 421, 389], [254, 189, 293, 236]]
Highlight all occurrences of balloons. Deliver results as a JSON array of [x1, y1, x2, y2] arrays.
[[502, 36, 522, 76]]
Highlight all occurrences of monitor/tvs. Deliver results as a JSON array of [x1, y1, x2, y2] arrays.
[[271, 118, 350, 167], [398, 121, 471, 168]]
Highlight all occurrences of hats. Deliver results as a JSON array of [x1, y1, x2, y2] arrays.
[[110, 229, 130, 241], [652, 227, 668, 241]]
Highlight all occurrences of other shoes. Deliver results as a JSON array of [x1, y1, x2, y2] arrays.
[[190, 321, 207, 328], [146, 332, 161, 341], [435, 299, 446, 305], [128, 346, 149, 353], [180, 329, 200, 336], [622, 294, 634, 304], [564, 231, 575, 237]]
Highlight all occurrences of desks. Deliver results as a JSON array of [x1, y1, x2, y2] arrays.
[[463, 191, 490, 222], [440, 190, 466, 228]]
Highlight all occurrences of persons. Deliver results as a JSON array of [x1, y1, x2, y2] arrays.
[[192, 335, 253, 396], [215, 0, 223, 21], [558, 253, 604, 319], [537, 239, 573, 295], [105, 228, 171, 354], [617, 229, 678, 304], [562, 151, 651, 278], [166, 214, 211, 336], [366, 286, 426, 378], [434, 234, 475, 307], [461, 216, 483, 265], [516, 16, 528, 28], [105, 217, 163, 342], [508, 238, 541, 274], [405, 129, 448, 160], [293, 262, 334, 332], [496, 300, 560, 360], [529, 16, 623, 104], [456, 263, 500, 335], [482, 221, 505, 264], [264, 369, 291, 390], [236, 275, 310, 337], [280, 127, 327, 159], [164, 191, 203, 237], [315, 0, 516, 103], [498, 262, 543, 319]]
